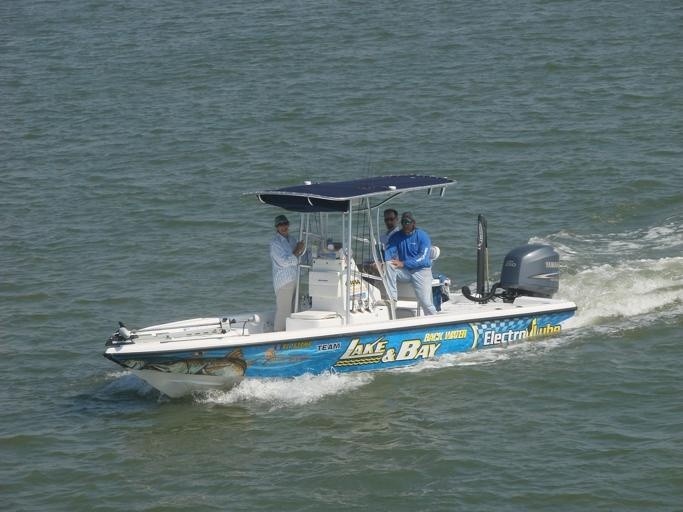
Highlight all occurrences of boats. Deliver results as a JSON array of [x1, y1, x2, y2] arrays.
[[102, 172, 579, 402]]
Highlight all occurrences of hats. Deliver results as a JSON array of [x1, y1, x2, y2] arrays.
[[402, 211, 415, 220], [274, 215, 289, 226]]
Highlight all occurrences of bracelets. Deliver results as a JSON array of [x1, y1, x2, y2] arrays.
[[295, 249, 301, 254]]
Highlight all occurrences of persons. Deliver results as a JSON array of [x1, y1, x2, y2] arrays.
[[361, 209, 401, 284], [269, 214, 304, 331], [382, 210, 437, 315]]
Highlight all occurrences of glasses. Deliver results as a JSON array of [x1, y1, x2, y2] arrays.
[[384, 217, 396, 221], [401, 220, 412, 225]]
